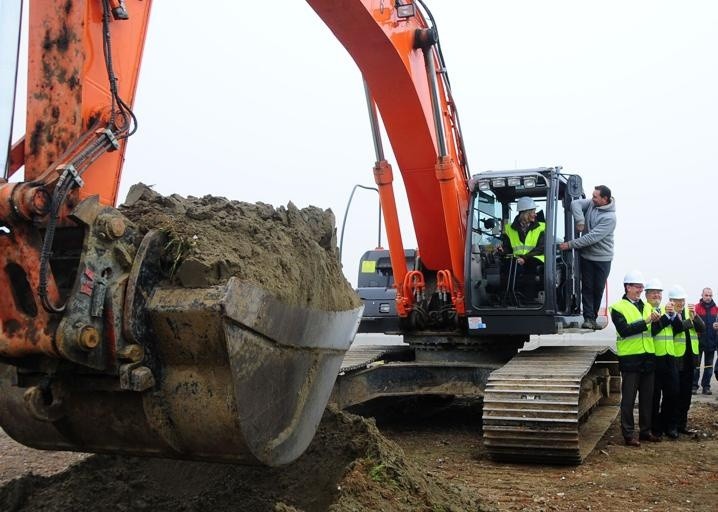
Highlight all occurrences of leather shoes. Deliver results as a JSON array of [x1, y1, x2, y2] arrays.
[[639, 428, 696, 442], [625, 437, 640, 446]]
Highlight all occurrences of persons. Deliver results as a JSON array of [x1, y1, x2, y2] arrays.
[[496, 197, 544, 302], [667, 285, 702, 440], [692, 287, 718, 393], [608, 270, 668, 447], [642, 275, 684, 442], [558, 183, 619, 330]]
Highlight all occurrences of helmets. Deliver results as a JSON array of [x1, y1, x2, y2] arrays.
[[644, 280, 663, 290], [517, 197, 539, 211], [669, 286, 685, 299], [623, 271, 643, 284]]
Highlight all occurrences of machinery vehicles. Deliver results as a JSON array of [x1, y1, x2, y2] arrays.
[[1, 0, 622, 471]]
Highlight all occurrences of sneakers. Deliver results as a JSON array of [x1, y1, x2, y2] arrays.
[[582, 318, 602, 329], [692, 386, 697, 394], [702, 388, 712, 395]]
